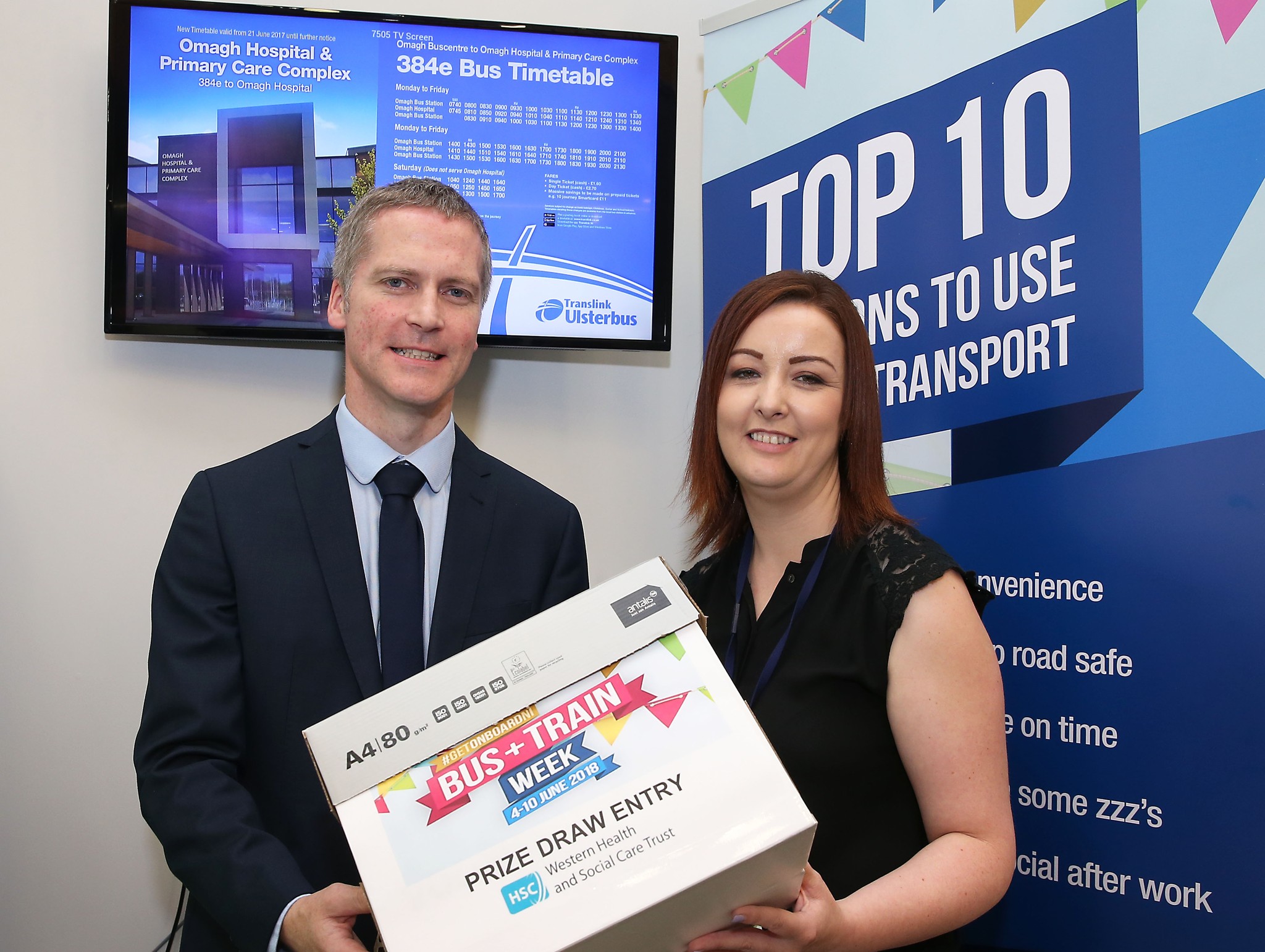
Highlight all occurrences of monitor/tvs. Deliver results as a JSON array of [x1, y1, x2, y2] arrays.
[[104, 0, 678, 354]]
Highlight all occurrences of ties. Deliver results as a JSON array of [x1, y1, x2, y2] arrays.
[[373, 462, 426, 692]]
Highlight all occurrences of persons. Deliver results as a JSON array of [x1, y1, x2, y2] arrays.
[[134, 178, 589, 952], [679, 270, 1016, 952]]
